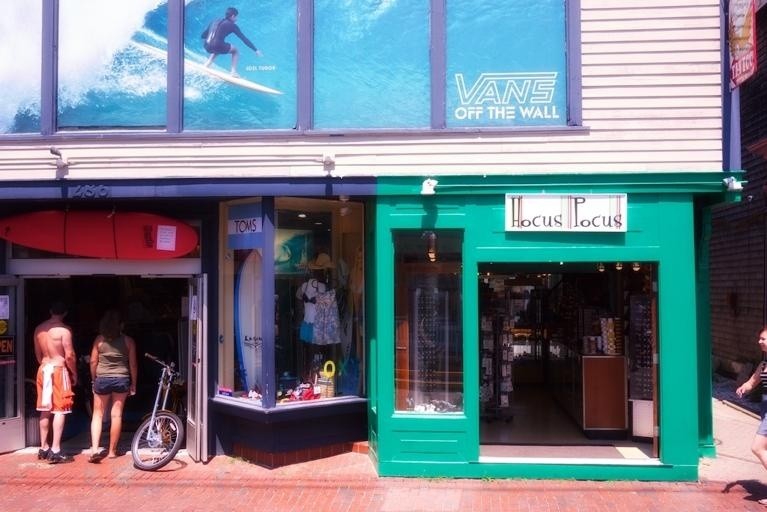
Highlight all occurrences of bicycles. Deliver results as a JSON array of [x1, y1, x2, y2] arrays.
[[131, 351, 187, 472]]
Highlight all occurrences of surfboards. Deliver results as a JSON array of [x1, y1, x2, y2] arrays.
[[0, 210, 199, 260], [234, 250, 262, 393], [130, 40, 282, 95]]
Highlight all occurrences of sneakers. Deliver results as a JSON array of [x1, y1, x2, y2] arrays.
[[38, 448, 73, 464], [88, 453, 101, 463]]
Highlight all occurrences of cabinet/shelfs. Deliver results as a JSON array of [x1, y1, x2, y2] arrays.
[[503, 276, 547, 385], [548, 338, 629, 440]]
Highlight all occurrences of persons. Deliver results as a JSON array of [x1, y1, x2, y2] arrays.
[[201, 4, 263, 79], [32, 296, 78, 464], [735, 324, 767, 508], [86, 306, 138, 463], [296, 274, 326, 373]]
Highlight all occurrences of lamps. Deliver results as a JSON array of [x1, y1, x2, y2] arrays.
[[420, 179, 438, 195], [423, 230, 439, 263], [596, 261, 640, 271], [721, 176, 744, 192]]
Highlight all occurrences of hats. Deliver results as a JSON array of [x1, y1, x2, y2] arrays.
[[308, 253, 336, 270]]
